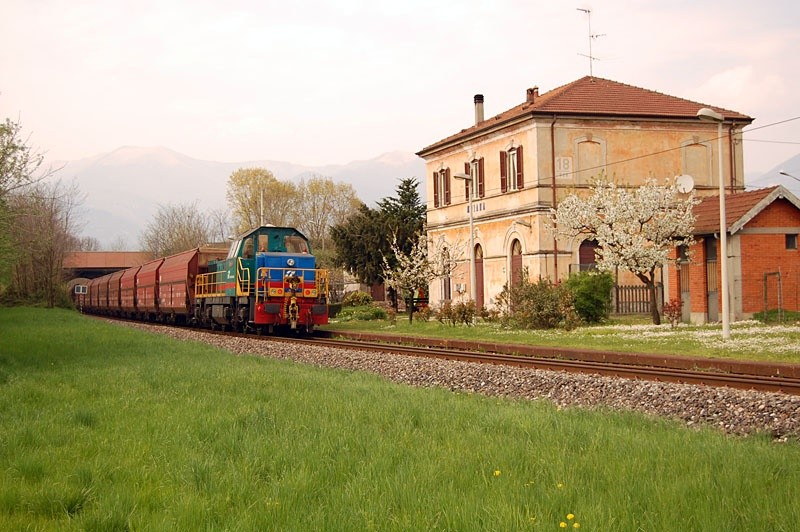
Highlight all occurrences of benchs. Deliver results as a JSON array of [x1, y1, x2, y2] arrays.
[[405, 297, 429, 312]]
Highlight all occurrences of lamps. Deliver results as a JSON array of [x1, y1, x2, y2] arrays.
[[516, 220, 531, 227]]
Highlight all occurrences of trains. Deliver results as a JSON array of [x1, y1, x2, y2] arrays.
[[66, 222, 330, 337]]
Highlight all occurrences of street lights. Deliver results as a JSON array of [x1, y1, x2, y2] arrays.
[[453, 172, 479, 326], [696, 107, 732, 340]]
